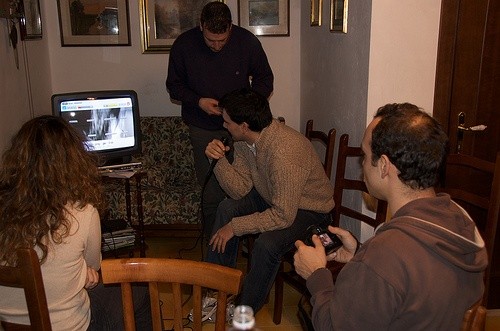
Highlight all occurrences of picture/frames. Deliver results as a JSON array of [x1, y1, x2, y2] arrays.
[[237, 0, 290, 37], [330, 0, 349, 34], [18, 0, 44, 40], [310, 0, 322, 27], [56, 0, 131, 48], [138, 0, 227, 55]]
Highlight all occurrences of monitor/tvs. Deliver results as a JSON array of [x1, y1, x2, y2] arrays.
[[52, 90, 142, 167]]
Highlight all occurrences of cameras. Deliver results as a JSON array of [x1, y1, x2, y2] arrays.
[[303, 224, 344, 256]]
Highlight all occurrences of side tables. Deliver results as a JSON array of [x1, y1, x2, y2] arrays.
[[97, 166, 149, 261]]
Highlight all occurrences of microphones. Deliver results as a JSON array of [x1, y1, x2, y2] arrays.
[[204, 136, 228, 181]]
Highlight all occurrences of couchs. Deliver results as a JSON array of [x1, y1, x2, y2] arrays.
[[95, 114, 286, 240]]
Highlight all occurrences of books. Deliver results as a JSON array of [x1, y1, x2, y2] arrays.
[[100, 218, 137, 250]]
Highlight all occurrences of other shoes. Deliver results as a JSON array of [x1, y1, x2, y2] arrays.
[[187, 286, 237, 328]]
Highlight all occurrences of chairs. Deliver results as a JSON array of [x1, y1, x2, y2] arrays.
[[0, 248, 60, 331], [245, 119, 389, 326], [434, 146, 500, 309], [99, 255, 245, 331]]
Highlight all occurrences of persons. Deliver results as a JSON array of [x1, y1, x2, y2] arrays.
[[294, 102, 488, 331], [189, 86, 337, 320], [0, 114, 154, 331], [165, 1, 274, 245]]
